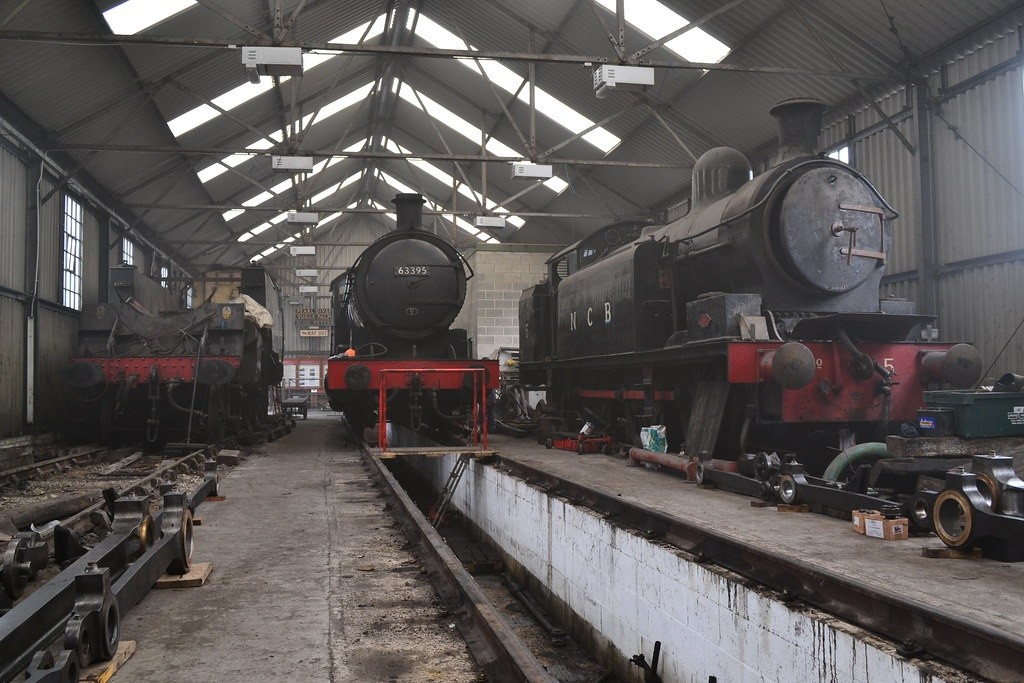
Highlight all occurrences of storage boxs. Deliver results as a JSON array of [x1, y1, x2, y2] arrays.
[[917, 407, 955, 437], [864, 516, 909, 541], [851, 509, 881, 535]]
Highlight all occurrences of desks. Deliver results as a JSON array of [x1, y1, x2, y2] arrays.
[[281, 397, 308, 420]]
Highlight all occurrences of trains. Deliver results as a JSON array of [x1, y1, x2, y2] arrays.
[[482, 94, 1024, 555], [50, 257, 301, 447], [320, 191, 502, 440]]
